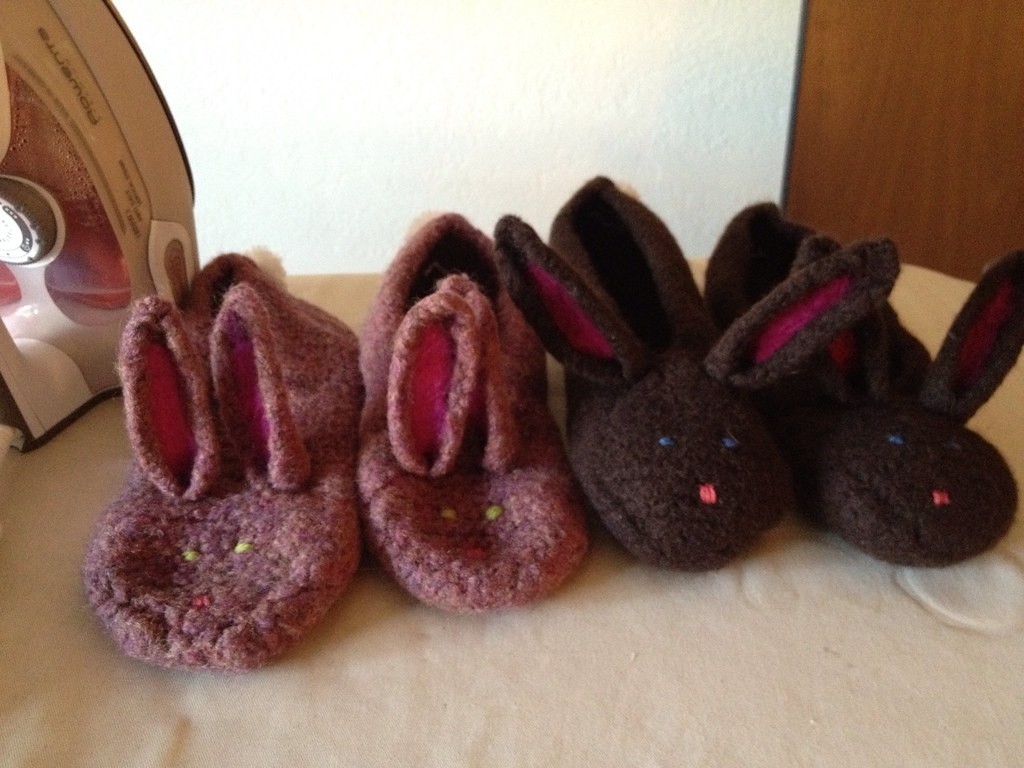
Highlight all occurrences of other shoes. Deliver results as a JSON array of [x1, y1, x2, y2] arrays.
[[359, 212, 594, 611], [495, 174, 895, 571], [84, 250, 359, 673], [705, 201, 1024, 568]]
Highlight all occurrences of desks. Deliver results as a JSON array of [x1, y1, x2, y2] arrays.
[[0, 255, 1024, 768]]
